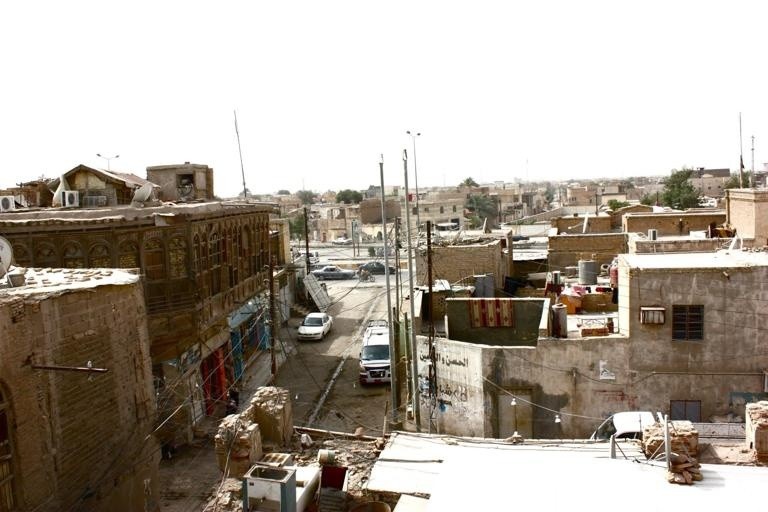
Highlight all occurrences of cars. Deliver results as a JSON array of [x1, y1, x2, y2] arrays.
[[310, 265, 356, 281], [297, 312, 333, 341], [359, 261, 396, 275]]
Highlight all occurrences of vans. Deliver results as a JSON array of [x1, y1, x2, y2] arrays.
[[590, 410, 657, 442], [359, 325, 391, 385]]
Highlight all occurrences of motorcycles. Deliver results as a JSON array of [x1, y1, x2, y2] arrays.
[[358, 272, 375, 283]]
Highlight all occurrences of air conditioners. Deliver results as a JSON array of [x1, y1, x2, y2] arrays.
[[551, 270, 561, 285], [648, 229, 657, 240], [0, 196, 15, 212], [61, 191, 79, 208]]
